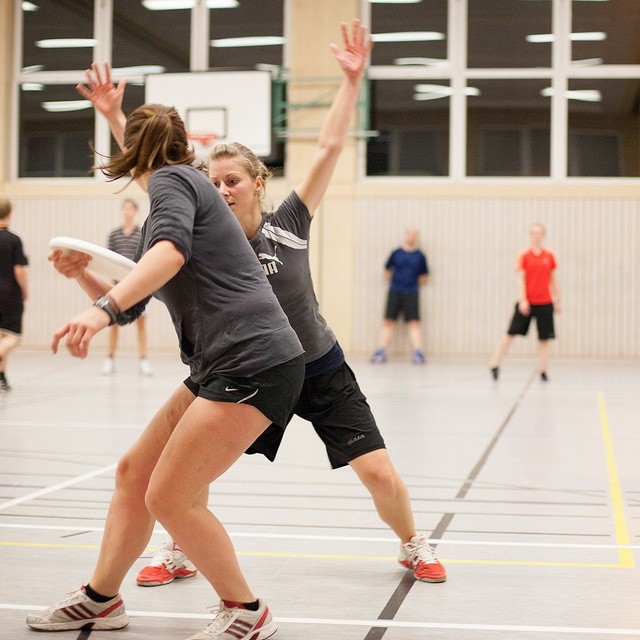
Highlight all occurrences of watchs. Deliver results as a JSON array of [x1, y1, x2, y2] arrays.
[[95, 297, 118, 326]]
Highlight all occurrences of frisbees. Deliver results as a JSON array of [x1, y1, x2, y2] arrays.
[[50, 237, 137, 282]]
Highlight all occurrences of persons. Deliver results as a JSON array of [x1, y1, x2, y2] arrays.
[[370, 227, 431, 364], [1, 198, 28, 391], [25, 102, 305, 640], [100, 199, 156, 379], [75, 19, 448, 588], [490, 222, 562, 381]]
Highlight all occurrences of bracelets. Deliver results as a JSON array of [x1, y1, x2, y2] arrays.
[[105, 292, 120, 316]]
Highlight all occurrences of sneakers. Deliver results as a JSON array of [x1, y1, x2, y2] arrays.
[[397, 533, 446, 583], [0, 372, 10, 391], [26, 585, 130, 631], [490, 367, 499, 379], [371, 348, 387, 364], [138, 358, 155, 377], [185, 598, 279, 640], [136, 540, 198, 587], [541, 372, 546, 381], [414, 348, 425, 365], [99, 357, 116, 375]]
